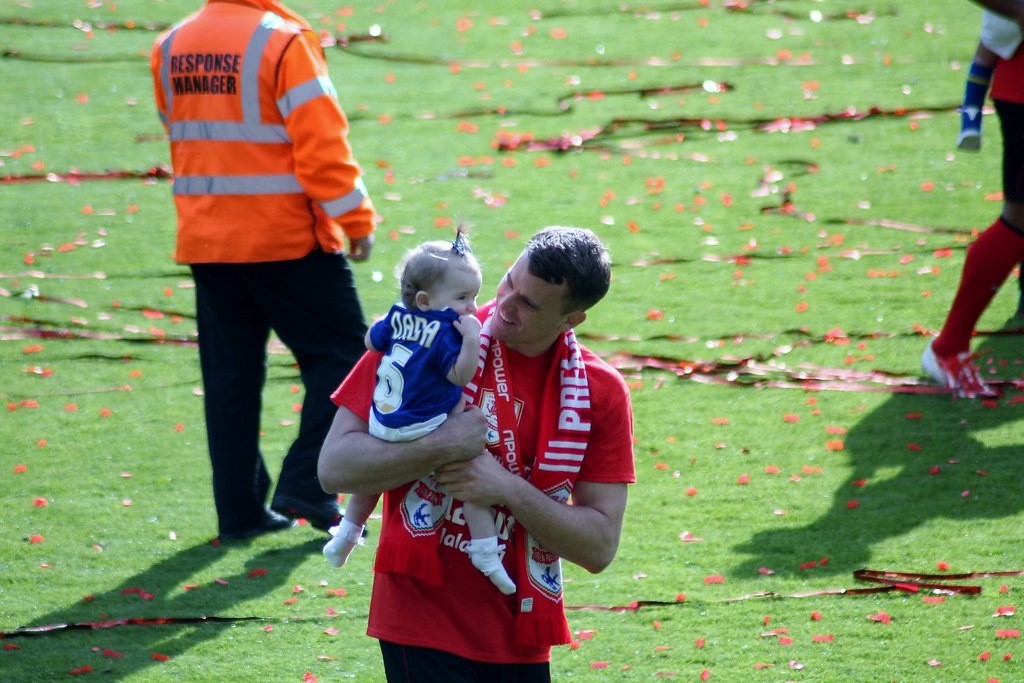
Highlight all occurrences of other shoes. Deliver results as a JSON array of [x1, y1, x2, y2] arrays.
[[957, 106, 982, 151], [273, 479, 367, 537], [219, 509, 295, 539]]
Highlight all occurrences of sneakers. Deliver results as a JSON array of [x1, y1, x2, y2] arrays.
[[923, 341, 998, 400]]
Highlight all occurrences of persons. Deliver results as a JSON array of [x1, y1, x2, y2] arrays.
[[149, 0, 376, 545], [322, 212, 517, 595], [919, 0, 1024, 401], [317, 224, 636, 683]]
